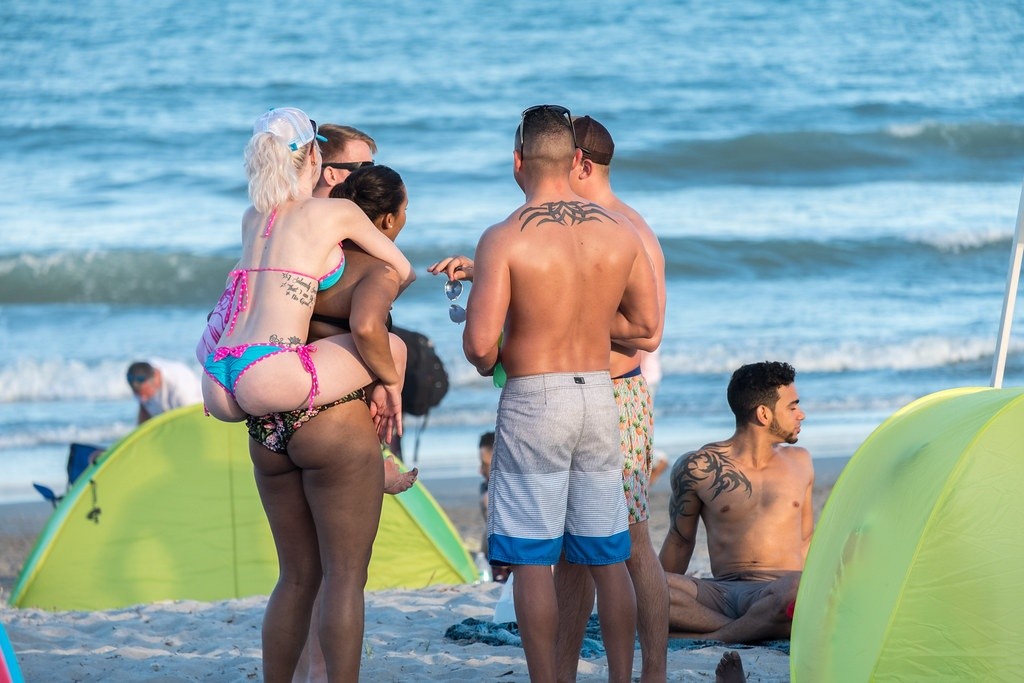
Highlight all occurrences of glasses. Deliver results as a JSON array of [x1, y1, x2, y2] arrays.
[[322, 162, 374, 172], [520, 104, 577, 161], [309, 118, 319, 155], [444, 265, 473, 325]]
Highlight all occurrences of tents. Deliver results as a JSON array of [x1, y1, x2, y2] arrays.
[[0, 617, 27, 683], [789, 387, 1023, 683], [5, 401, 482, 613]]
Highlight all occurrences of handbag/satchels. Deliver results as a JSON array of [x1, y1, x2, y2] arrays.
[[385, 310, 450, 416]]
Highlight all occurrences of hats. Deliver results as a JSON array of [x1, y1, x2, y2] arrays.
[[253, 107, 328, 151], [573, 115, 614, 165]]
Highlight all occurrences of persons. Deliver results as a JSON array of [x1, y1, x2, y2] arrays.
[[126, 357, 204, 425], [715, 650, 747, 683], [426, 103, 668, 683], [658, 361, 817, 646], [195, 106, 420, 683]]
